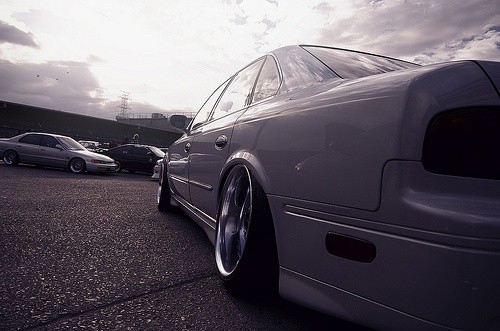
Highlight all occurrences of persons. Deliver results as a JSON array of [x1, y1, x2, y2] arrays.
[[110, 137, 130, 149]]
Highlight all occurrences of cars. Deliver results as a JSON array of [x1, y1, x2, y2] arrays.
[[157, 43, 500, 331], [115, 146, 165, 172], [0, 132, 116, 174], [100, 144, 147, 169]]
